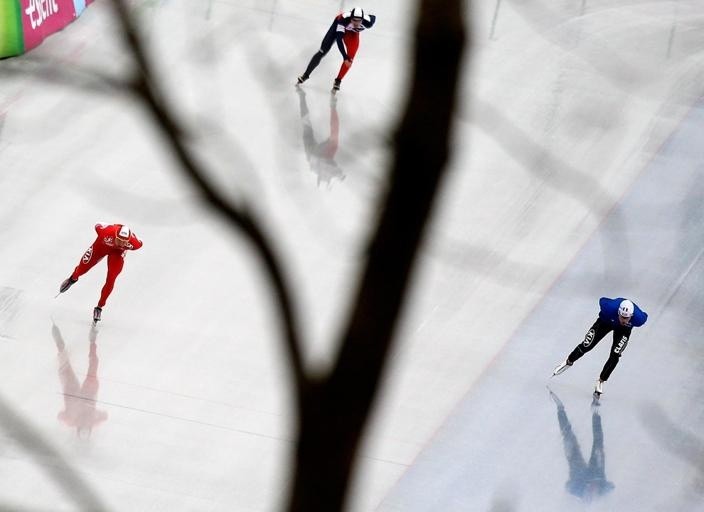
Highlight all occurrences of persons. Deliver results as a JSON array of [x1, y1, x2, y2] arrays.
[[59, 219, 143, 324], [297, 8, 376, 94], [547, 294, 647, 395]]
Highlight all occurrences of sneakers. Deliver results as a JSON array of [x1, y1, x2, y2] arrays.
[[60, 276, 78, 293], [94, 306, 102, 321], [334, 78, 342, 90], [554, 360, 574, 375], [298, 74, 309, 82], [595, 381, 604, 394]]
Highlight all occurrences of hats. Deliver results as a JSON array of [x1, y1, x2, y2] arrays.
[[352, 7, 364, 20]]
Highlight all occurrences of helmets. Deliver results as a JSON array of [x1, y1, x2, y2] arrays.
[[116, 225, 131, 241], [619, 300, 635, 318]]
